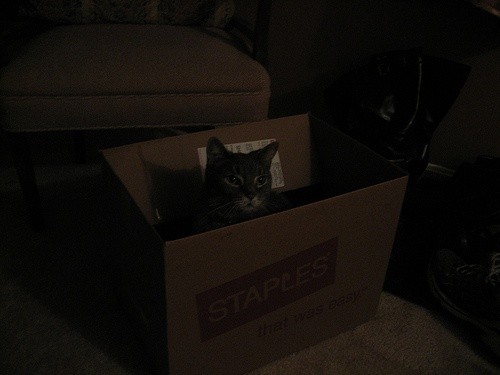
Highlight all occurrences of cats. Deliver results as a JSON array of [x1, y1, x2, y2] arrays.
[[191, 135, 294, 235]]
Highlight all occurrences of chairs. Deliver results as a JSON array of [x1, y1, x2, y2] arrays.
[[0, 0, 270, 234]]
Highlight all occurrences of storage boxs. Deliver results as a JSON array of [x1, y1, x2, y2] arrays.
[[99, 112, 409, 375]]
